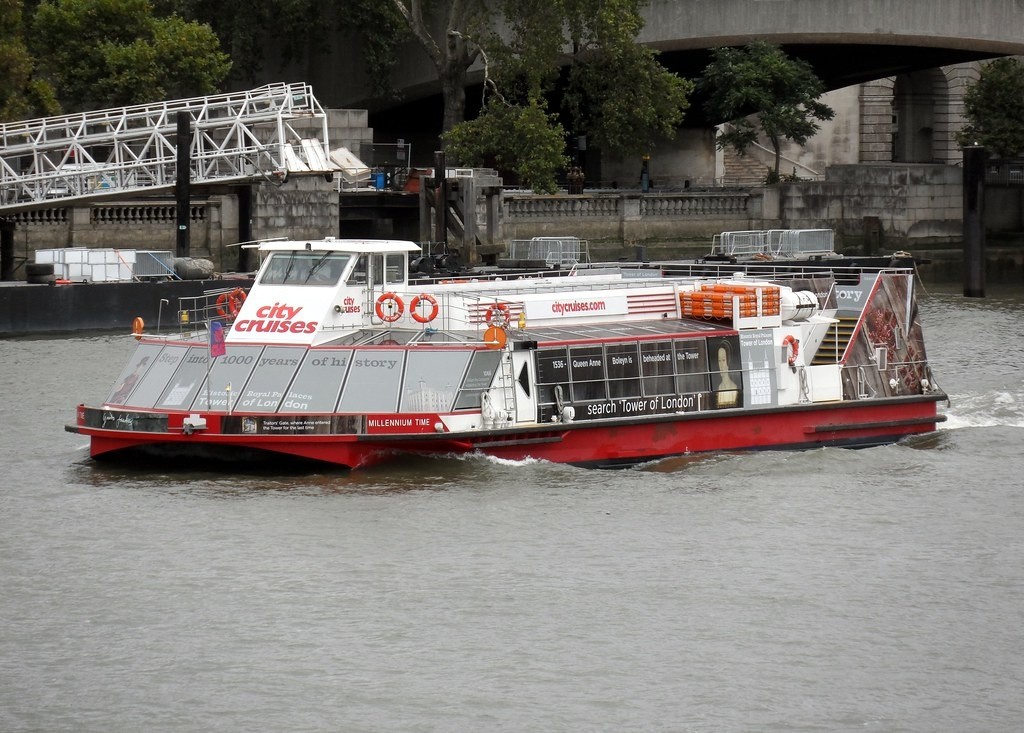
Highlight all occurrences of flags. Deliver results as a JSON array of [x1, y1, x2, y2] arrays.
[[211, 321, 226, 357]]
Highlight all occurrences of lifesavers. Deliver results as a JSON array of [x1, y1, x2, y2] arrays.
[[782, 335, 799, 364], [214, 287, 247, 322], [485, 304, 510, 331], [409, 295, 438, 322], [375, 292, 405, 323]]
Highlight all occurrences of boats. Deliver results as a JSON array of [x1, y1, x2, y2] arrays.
[[660, 227, 933, 286], [61, 237, 949, 472]]
